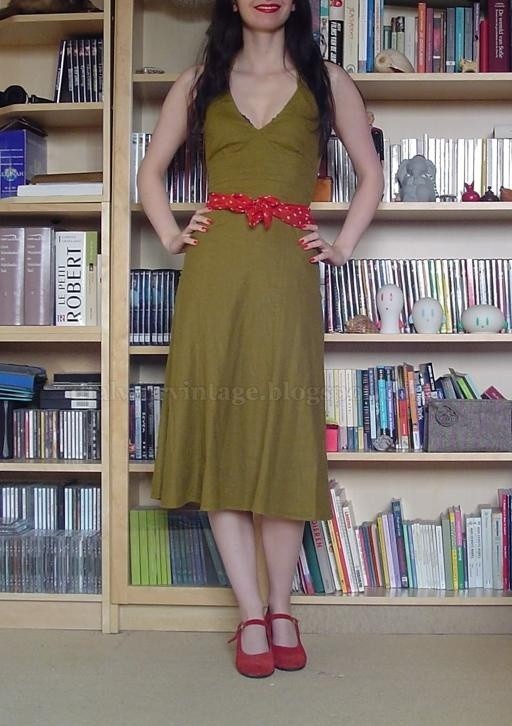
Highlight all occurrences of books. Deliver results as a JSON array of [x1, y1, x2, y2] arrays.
[[323, 126, 511, 203], [0, 363, 101, 460], [1, 482, 101, 594], [294, 478, 512, 593], [129, 384, 165, 459], [17, 171, 103, 196], [320, 260, 510, 332], [311, 0, 512, 74], [54, 40, 103, 103], [1, 223, 103, 327], [327, 361, 507, 450], [129, 269, 181, 347], [130, 132, 208, 204], [130, 506, 231, 587]]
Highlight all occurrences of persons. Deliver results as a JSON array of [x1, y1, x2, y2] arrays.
[[138, 0, 384, 679], [367, 110, 384, 166]]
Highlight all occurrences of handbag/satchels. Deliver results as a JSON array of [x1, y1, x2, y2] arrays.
[[422, 397, 512, 452]]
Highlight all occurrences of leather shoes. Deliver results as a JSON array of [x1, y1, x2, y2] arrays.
[[228, 618, 276, 678], [265, 605, 307, 672]]
